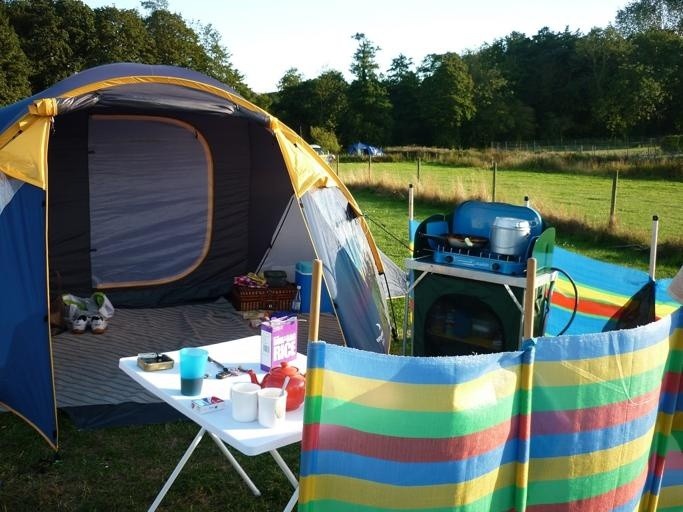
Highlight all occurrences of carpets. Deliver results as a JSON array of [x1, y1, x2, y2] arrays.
[[48, 297, 349, 413]]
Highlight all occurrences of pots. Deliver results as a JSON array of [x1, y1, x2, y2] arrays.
[[419, 230, 489, 250], [488, 215, 531, 255]]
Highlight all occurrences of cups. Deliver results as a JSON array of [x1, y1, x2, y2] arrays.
[[178, 347, 209, 397], [257, 387, 287, 428], [230, 382, 261, 423]]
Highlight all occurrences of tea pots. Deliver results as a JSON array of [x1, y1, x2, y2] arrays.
[[245, 362, 306, 412]]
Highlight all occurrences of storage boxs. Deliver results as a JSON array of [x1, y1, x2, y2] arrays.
[[294, 267, 333, 315], [262, 269, 288, 289]]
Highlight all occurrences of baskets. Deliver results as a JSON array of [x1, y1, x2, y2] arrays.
[[231, 279, 297, 311]]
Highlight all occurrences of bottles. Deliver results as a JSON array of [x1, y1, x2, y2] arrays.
[[428, 303, 459, 338], [291, 285, 302, 313]]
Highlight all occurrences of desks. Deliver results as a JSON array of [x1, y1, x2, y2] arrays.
[[117, 334, 313, 512]]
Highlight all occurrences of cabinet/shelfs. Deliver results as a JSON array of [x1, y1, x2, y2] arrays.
[[397, 253, 562, 359]]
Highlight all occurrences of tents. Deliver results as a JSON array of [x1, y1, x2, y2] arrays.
[[349, 140, 383, 157], [0, 62, 396, 453]]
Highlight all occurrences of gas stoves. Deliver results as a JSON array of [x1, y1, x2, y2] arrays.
[[430, 242, 524, 276]]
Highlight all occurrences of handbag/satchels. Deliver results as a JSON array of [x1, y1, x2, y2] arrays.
[[61, 292, 114, 329]]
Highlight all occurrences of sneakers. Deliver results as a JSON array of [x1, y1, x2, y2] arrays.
[[72, 315, 108, 334]]
